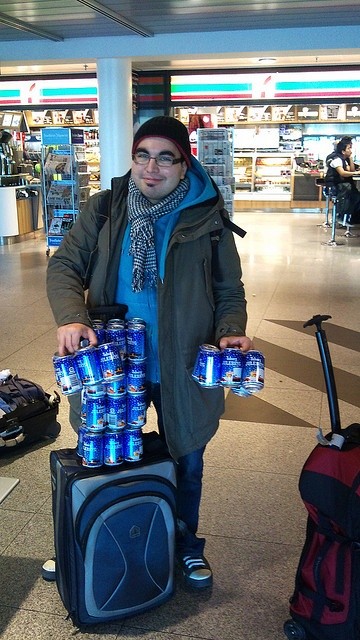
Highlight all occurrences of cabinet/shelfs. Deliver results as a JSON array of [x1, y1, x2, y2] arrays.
[[235, 150, 295, 201], [20, 108, 101, 192], [196, 126, 235, 221], [41, 144, 91, 255]]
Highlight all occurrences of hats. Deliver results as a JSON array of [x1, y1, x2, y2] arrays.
[[132, 115, 193, 169]]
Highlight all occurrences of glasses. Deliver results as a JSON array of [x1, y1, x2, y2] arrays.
[[133, 151, 183, 167]]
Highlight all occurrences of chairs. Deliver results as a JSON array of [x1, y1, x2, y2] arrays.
[[315, 178, 355, 244]]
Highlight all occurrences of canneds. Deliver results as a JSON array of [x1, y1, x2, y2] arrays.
[[192, 343, 265, 398], [54, 318, 146, 468]]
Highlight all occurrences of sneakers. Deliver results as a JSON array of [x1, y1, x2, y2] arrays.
[[338, 217, 355, 228], [41, 556, 57, 581], [176, 554, 213, 589]]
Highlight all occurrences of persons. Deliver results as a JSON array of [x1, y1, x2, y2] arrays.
[[324, 140, 360, 227], [42, 116, 251, 590], [340, 136, 359, 171], [326, 141, 340, 164]]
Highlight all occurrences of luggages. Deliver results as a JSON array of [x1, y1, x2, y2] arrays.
[[0, 368, 62, 459], [50, 304, 178, 629], [283, 313, 359, 640]]
